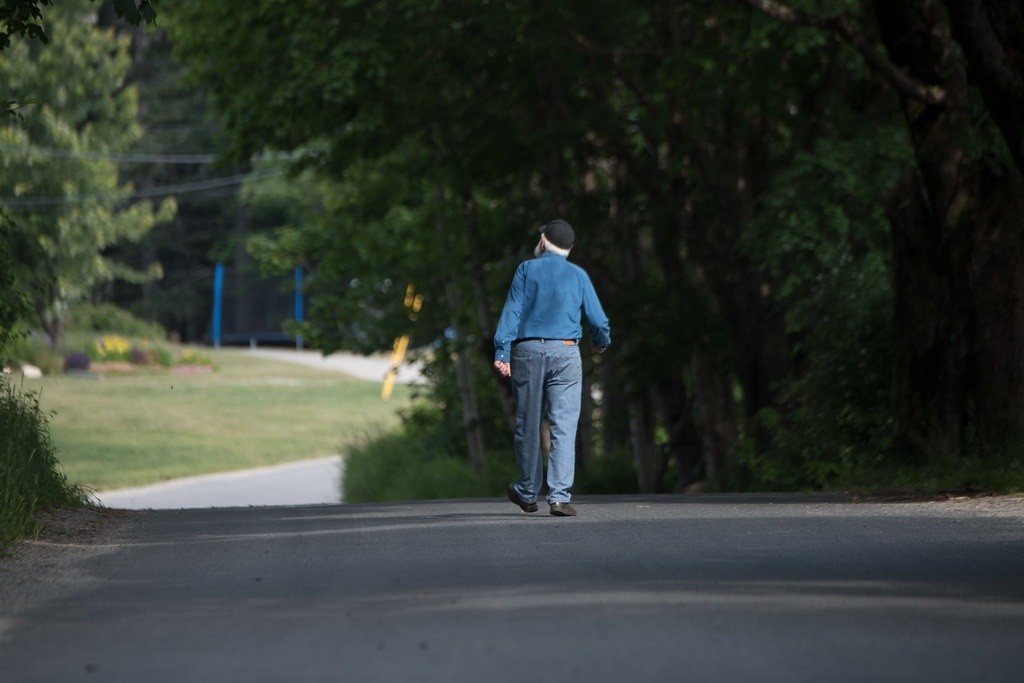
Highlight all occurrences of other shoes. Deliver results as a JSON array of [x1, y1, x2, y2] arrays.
[[550, 503, 577, 516], [508, 482, 538, 513]]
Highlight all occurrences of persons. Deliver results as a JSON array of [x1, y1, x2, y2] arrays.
[[492, 218, 611, 516]]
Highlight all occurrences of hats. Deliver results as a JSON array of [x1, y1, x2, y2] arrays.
[[538, 219, 575, 249]]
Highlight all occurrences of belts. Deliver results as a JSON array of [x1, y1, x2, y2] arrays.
[[513, 337, 576, 346]]
